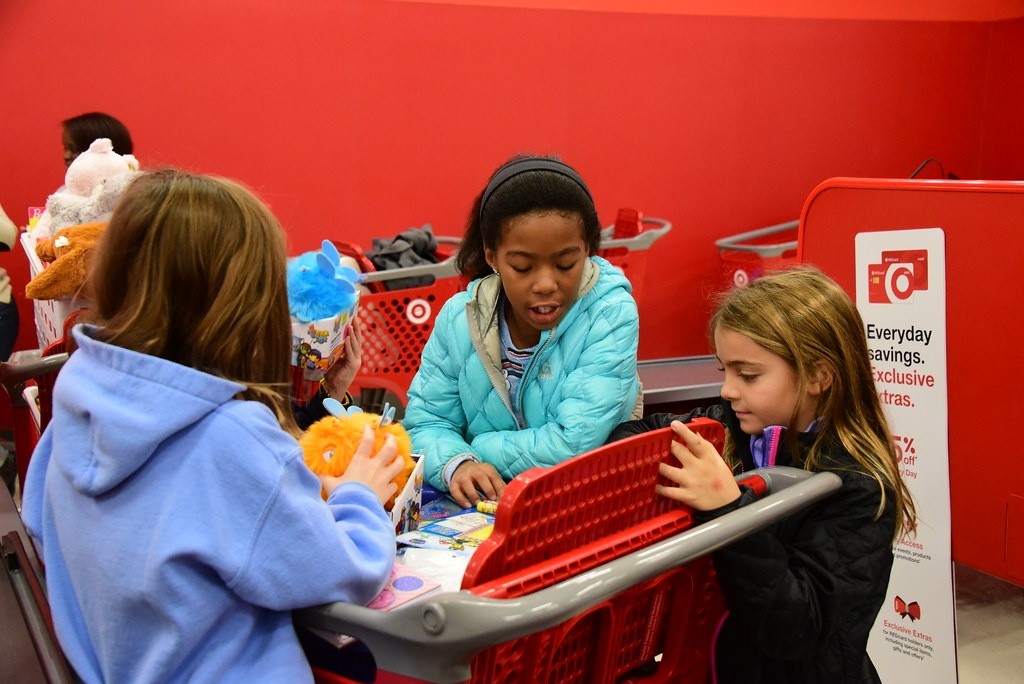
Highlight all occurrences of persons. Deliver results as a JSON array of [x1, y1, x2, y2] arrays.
[[400, 152, 643, 507], [62, 112, 133, 172], [20, 167, 405, 684], [657, 265, 917, 684]]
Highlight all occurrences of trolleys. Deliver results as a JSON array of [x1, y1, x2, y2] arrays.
[[0, 165, 1024, 684]]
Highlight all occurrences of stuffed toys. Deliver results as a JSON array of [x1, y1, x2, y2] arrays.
[[284, 239, 360, 321], [296, 396, 416, 509], [45, 168, 145, 235], [24, 222, 110, 301], [26, 137, 139, 246]]
